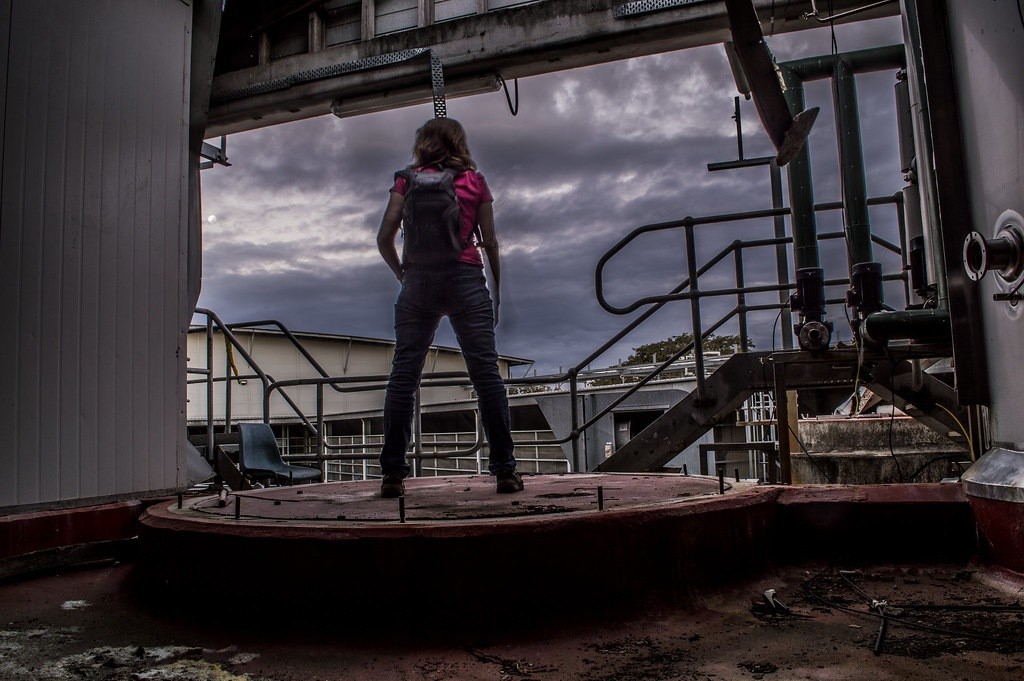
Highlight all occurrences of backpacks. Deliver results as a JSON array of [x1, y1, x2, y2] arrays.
[[394, 164, 484, 267]]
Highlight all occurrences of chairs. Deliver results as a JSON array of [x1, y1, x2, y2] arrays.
[[237, 422, 322, 491]]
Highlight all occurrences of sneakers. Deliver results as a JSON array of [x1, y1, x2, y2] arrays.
[[496, 468, 524, 493], [381, 472, 405, 498]]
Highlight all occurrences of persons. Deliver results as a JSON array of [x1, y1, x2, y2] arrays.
[[374, 118, 525, 499]]
